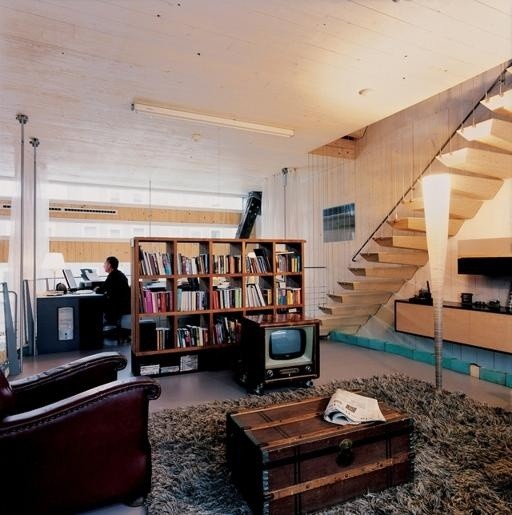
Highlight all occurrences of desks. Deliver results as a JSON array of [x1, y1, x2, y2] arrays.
[[35, 289, 100, 354]]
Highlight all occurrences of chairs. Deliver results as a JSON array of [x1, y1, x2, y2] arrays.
[[0, 346, 162, 514], [117, 314, 131, 347]]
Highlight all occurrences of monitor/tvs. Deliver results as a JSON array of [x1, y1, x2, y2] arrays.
[[265, 326, 313, 370], [62, 268, 77, 289]]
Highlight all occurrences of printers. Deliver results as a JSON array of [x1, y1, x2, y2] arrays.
[[79, 269, 105, 288]]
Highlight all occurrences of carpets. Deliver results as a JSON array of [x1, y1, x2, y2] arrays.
[[147, 370, 511, 515]]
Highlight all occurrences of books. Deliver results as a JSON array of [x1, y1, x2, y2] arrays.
[[138, 246, 301, 353]]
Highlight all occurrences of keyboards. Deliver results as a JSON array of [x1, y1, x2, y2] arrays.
[[76, 290, 93, 294]]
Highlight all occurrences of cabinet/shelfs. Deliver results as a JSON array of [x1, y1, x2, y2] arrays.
[[131, 235, 309, 378]]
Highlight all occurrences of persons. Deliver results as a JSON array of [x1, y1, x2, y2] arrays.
[[94, 256, 133, 344]]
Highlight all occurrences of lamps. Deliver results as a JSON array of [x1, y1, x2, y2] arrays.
[[419, 170, 454, 394], [41, 253, 65, 296], [129, 101, 294, 140]]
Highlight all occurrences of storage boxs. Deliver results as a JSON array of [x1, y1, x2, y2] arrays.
[[226, 386, 415, 514]]
